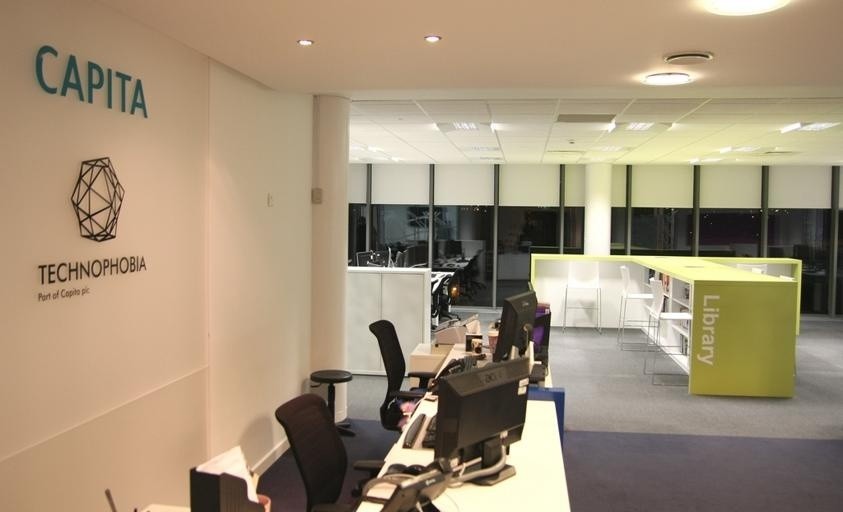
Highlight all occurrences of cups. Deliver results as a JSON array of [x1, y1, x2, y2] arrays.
[[470, 339, 483, 355], [488, 331, 499, 352]]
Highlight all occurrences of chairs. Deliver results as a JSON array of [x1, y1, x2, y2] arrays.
[[369, 319, 435, 432], [562, 261, 603, 335], [617, 266, 658, 351], [439, 268, 462, 320], [274, 393, 384, 512], [643, 280, 691, 386], [460, 249, 486, 301]]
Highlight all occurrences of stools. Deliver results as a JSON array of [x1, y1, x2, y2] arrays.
[[310, 369, 355, 438]]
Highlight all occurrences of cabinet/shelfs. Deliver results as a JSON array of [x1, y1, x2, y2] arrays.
[[530, 254, 802, 398], [344, 267, 431, 377]]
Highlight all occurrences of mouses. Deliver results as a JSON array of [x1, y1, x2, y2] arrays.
[[402, 464, 426, 475]]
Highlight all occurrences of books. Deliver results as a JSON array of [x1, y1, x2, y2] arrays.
[[648, 269, 690, 355]]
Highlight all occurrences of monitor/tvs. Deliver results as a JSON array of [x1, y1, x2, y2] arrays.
[[493, 291, 538, 361], [355, 239, 462, 266], [379, 464, 458, 512], [433, 356, 531, 487]]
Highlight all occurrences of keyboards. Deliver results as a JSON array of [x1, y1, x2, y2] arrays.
[[462, 355, 477, 373], [422, 411, 438, 447]]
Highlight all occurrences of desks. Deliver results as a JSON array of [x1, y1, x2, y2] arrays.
[[431, 271, 456, 294], [432, 343, 553, 388], [354, 391, 572, 512], [432, 257, 473, 270], [431, 272, 453, 318]]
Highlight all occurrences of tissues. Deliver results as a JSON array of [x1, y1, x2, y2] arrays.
[[466, 320, 483, 352]]
